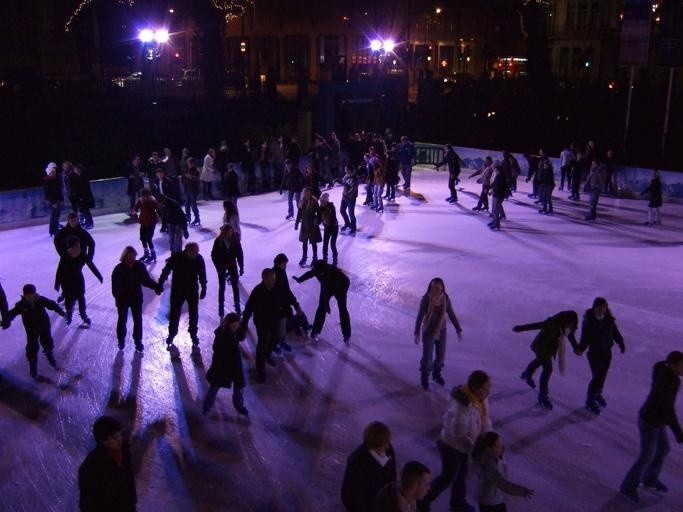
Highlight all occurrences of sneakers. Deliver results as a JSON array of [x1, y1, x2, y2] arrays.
[[642, 478, 669, 492], [235, 405, 250, 416], [521, 370, 537, 389], [585, 400, 600, 411], [432, 370, 446, 386], [538, 396, 553, 409], [420, 373, 430, 390], [619, 485, 641, 503]]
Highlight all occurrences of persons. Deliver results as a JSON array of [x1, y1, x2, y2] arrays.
[[417, 370, 490, 512], [369, 146, 379, 156], [302, 163, 321, 198], [584, 167, 602, 222], [54, 237, 103, 325], [281, 158, 303, 218], [151, 168, 174, 233], [273, 136, 284, 189], [312, 135, 320, 163], [319, 193, 339, 267], [603, 151, 614, 194], [0, 285, 12, 331], [575, 297, 625, 413], [385, 150, 399, 200], [399, 136, 416, 190], [558, 147, 573, 192], [127, 156, 144, 218], [568, 162, 580, 201], [112, 247, 163, 350], [619, 351, 683, 505], [363, 153, 373, 205], [370, 134, 384, 157], [54, 212, 95, 303], [288, 137, 303, 170], [211, 225, 244, 321], [336, 165, 357, 235], [539, 160, 556, 214], [331, 132, 341, 180], [299, 260, 350, 341], [79, 415, 137, 512], [7, 285, 65, 377], [223, 162, 239, 209], [62, 161, 95, 228], [161, 148, 176, 176], [373, 461, 431, 512], [146, 152, 160, 196], [473, 432, 535, 512], [513, 311, 578, 408], [221, 200, 241, 282], [522, 152, 541, 198], [0, 283, 10, 329], [341, 422, 397, 512], [272, 254, 301, 354], [502, 151, 513, 195], [177, 148, 190, 175], [43, 161, 64, 235], [415, 278, 461, 390], [176, 173, 201, 226], [156, 191, 189, 264], [488, 167, 509, 229], [199, 149, 216, 200], [240, 138, 257, 193], [155, 243, 207, 344], [202, 313, 250, 415], [317, 137, 334, 189], [491, 160, 511, 220], [639, 169, 662, 226], [131, 188, 160, 263], [240, 269, 291, 384], [434, 143, 460, 203], [294, 189, 321, 268], [259, 141, 272, 192], [469, 157, 493, 212], [352, 133, 361, 144], [371, 157, 384, 212]]
